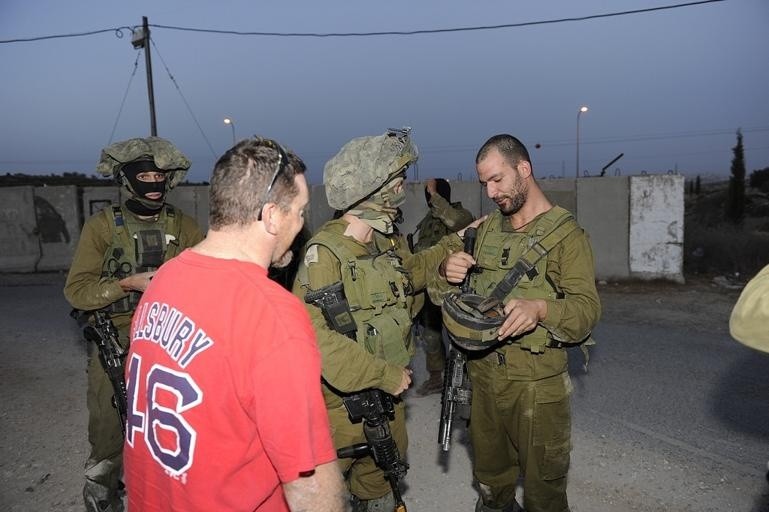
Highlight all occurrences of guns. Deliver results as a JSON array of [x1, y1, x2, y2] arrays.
[[305, 281, 410, 512], [71, 307, 128, 441], [438, 227, 484, 451]]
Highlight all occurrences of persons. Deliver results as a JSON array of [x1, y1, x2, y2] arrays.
[[406, 177, 475, 398], [122, 137, 346, 511], [290, 128, 488, 512], [61, 135, 204, 512], [425, 132, 603, 511]]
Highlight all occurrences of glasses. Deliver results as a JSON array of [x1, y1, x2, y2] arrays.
[[252, 134, 289, 220]]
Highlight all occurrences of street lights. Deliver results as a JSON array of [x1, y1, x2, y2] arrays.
[[577, 107, 590, 178], [224, 118, 237, 146]]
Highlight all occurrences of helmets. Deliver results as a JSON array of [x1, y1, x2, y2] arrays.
[[322, 129, 419, 211], [442, 292, 508, 353]]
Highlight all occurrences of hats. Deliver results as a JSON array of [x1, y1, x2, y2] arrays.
[[425, 178, 452, 207]]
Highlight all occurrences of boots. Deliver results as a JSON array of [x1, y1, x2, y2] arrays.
[[413, 372, 444, 398]]
